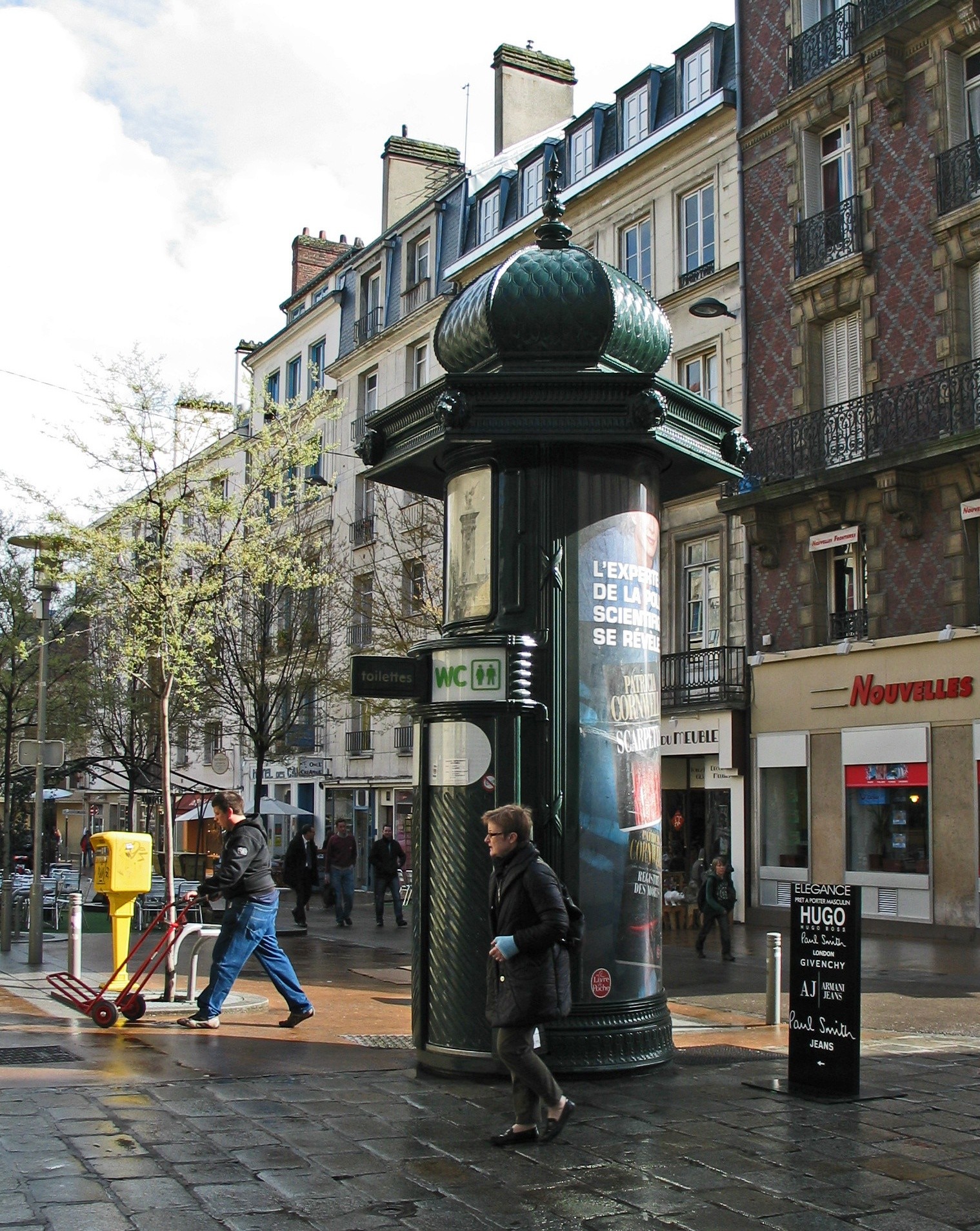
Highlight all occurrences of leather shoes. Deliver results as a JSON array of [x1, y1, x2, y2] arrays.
[[493, 1126, 539, 1146], [537, 1098, 575, 1144]]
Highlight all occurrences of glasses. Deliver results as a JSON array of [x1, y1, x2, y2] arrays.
[[487, 832, 504, 838]]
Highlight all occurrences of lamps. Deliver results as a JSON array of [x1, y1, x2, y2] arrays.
[[303, 473, 338, 493], [837, 639, 876, 656], [319, 779, 340, 790], [689, 297, 738, 320], [669, 713, 700, 728], [938, 624, 978, 642], [750, 651, 787, 666]]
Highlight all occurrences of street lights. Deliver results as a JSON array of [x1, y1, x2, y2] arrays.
[[6, 532, 89, 968]]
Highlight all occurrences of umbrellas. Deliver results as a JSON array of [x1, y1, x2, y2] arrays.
[[175, 796, 313, 821]]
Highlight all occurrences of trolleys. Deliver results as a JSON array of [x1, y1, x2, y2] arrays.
[[44, 894, 211, 1028]]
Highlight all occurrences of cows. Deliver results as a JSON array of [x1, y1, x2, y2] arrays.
[[664, 889, 686, 907]]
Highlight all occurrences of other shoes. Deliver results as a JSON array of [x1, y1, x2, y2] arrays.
[[176, 1015, 220, 1029], [297, 920, 308, 927], [344, 918, 352, 925], [722, 954, 735, 960], [377, 922, 383, 926], [279, 1007, 314, 1028], [398, 921, 407, 926], [338, 921, 344, 926], [695, 945, 705, 958]]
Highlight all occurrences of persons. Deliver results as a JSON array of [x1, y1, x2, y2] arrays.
[[694, 857, 736, 961], [80, 830, 94, 868], [52, 823, 62, 862], [324, 819, 357, 927], [369, 824, 407, 927], [478, 805, 577, 1147], [283, 823, 319, 927], [322, 830, 334, 851], [178, 791, 315, 1029]]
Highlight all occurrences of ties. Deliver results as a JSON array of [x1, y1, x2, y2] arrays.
[[305, 842, 310, 864]]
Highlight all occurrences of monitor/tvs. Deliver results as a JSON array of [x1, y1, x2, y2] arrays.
[[891, 832, 907, 849], [892, 809, 907, 826]]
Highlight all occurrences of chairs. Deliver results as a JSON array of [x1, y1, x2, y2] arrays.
[[398, 869, 412, 906], [662, 871, 704, 930], [0, 868, 203, 930]]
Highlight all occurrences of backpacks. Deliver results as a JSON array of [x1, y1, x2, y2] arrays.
[[522, 859, 585, 951], [696, 875, 717, 913]]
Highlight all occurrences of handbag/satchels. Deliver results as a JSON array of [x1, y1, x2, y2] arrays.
[[323, 881, 337, 908]]
